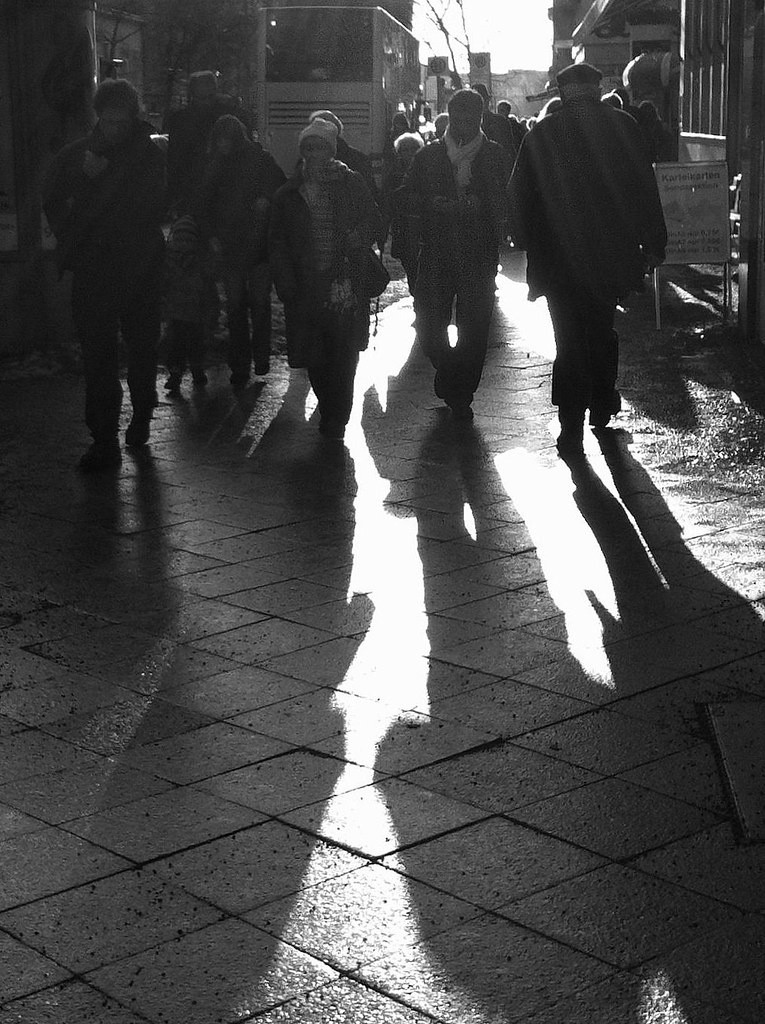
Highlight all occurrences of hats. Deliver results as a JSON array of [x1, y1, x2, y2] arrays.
[[556, 63, 602, 87], [298, 118, 338, 158], [309, 110, 342, 134], [167, 215, 201, 245]]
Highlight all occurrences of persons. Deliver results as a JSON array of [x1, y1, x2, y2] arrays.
[[375, 64, 672, 449], [154, 67, 386, 437], [38, 77, 169, 472]]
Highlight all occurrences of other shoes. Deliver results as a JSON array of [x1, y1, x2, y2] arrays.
[[317, 397, 346, 437], [230, 369, 249, 384], [589, 390, 621, 426], [125, 408, 151, 445], [78, 437, 122, 473], [193, 368, 207, 386], [255, 354, 269, 375], [434, 348, 472, 419], [557, 418, 584, 445], [164, 376, 182, 389]]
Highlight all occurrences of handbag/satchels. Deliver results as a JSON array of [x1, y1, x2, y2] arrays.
[[345, 169, 390, 297]]
